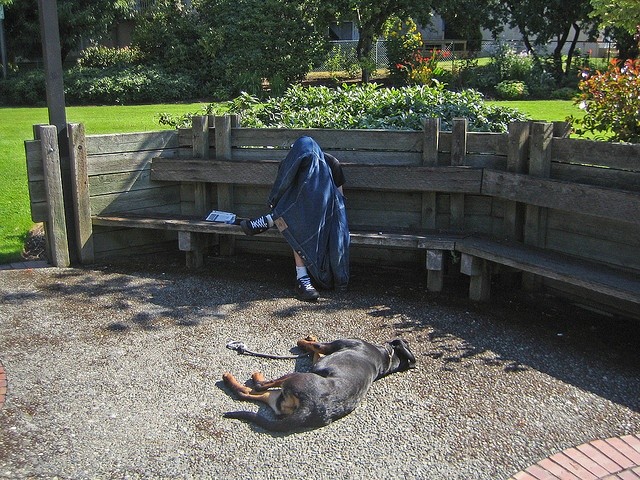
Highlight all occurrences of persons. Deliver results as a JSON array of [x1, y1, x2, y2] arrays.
[[241, 137, 345, 301]]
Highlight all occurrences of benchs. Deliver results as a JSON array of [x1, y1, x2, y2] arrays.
[[455, 230, 640, 305], [90, 211, 476, 291]]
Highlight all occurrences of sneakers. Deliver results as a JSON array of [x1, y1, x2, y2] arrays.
[[240, 215, 269, 236], [294, 276, 320, 300]]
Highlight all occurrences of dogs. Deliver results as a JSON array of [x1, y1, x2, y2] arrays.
[[222, 335, 416, 433]]
